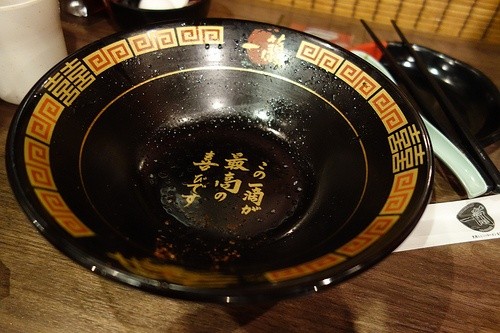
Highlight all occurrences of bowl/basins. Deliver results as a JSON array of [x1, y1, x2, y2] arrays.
[[110, 0, 210, 27], [5, 18, 434, 302], [374, 41, 500, 151]]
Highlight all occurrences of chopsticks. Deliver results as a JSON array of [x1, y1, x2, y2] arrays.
[[360, 18, 500, 195]]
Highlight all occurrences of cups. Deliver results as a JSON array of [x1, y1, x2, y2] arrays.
[[0, 0, 68, 105]]
